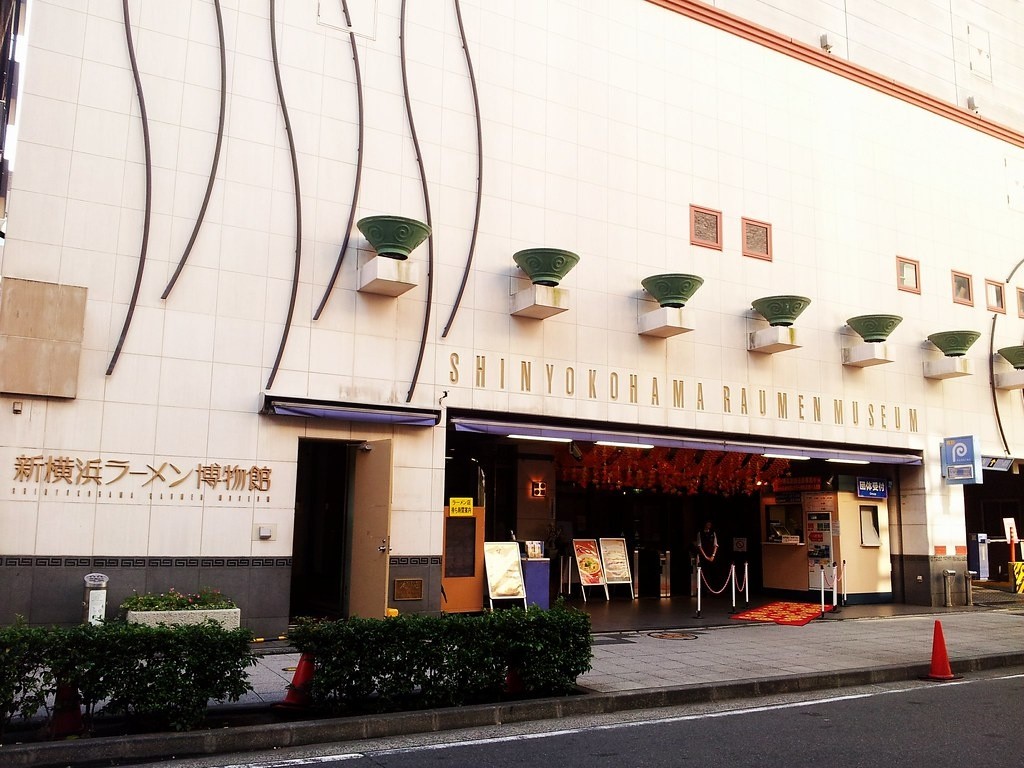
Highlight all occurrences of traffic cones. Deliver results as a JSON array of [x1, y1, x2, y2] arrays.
[[282, 652, 317, 706], [926, 618, 956, 680]]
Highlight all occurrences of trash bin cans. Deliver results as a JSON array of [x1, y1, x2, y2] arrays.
[[968, 533, 989, 580], [84, 572, 110, 626]]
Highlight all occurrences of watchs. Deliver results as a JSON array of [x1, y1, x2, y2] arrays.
[[712, 553, 715, 557]]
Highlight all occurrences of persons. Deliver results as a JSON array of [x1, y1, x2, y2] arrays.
[[697, 519, 719, 599]]
